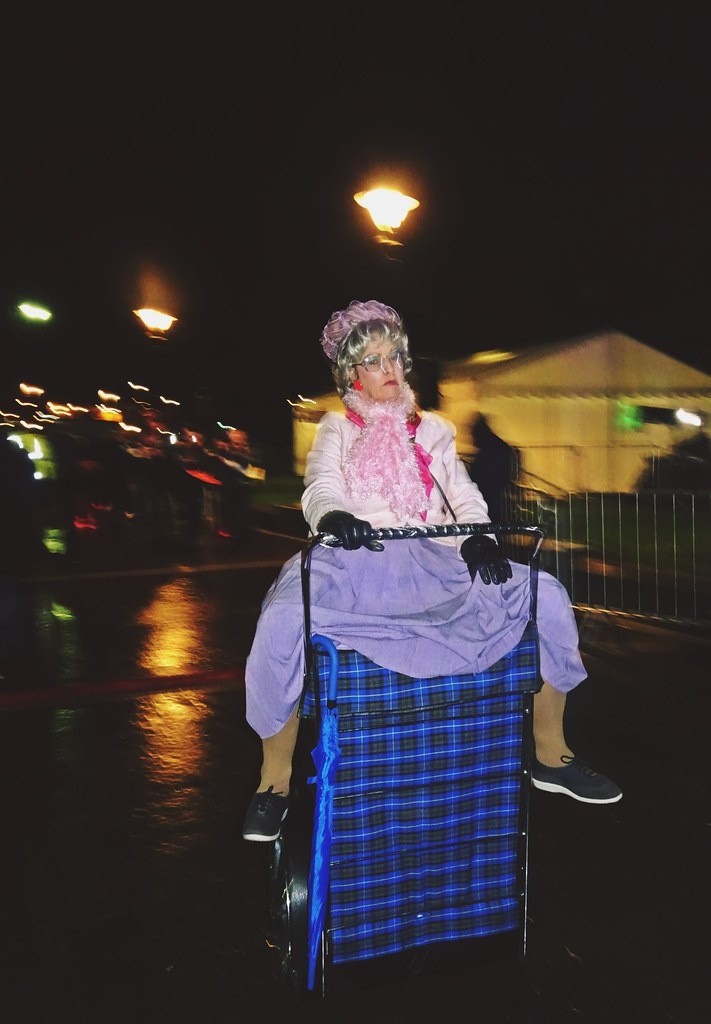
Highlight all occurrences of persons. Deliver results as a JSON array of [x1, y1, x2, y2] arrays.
[[243, 299, 623, 843]]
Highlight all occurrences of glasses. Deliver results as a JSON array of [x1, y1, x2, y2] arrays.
[[349, 349, 407, 373]]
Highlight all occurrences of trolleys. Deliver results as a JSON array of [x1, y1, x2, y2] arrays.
[[267, 525, 550, 1001]]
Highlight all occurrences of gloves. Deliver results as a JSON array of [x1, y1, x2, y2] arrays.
[[317, 509, 385, 552], [461, 535, 513, 585]]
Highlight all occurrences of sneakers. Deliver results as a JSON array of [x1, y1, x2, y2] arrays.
[[242, 785, 290, 842], [532, 754, 623, 805]]
